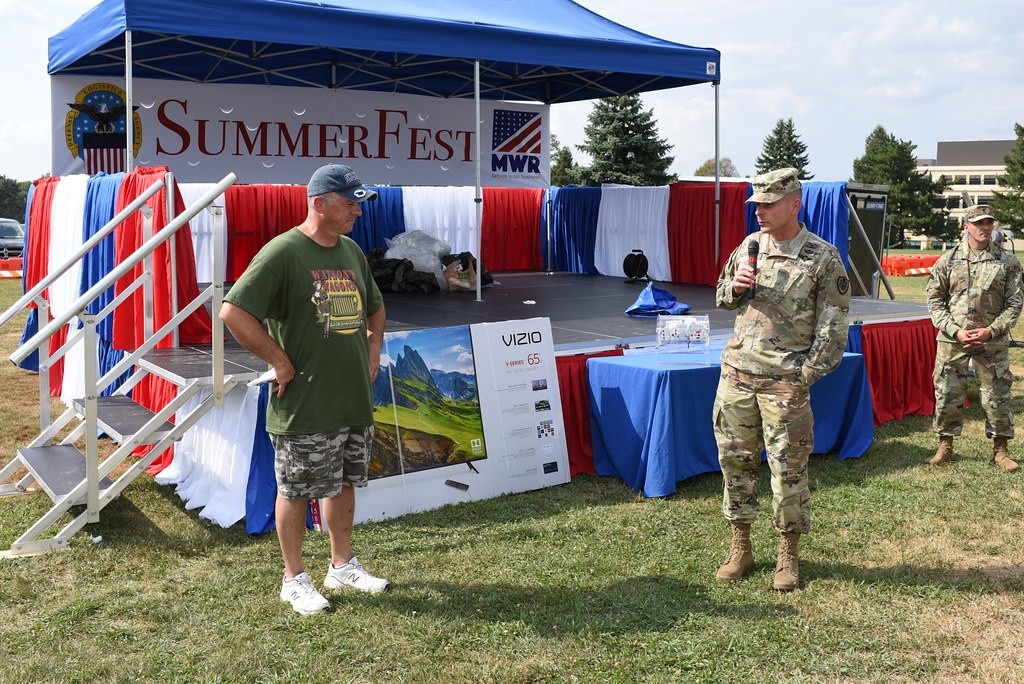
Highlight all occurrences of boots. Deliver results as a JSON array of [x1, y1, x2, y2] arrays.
[[923, 436, 953, 465], [716, 521, 753, 580], [773, 532, 800, 590], [993, 438, 1018, 472]]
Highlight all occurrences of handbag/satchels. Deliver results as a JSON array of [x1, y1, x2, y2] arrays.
[[444, 258, 478, 293]]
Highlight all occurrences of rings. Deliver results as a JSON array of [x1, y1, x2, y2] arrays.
[[370, 371, 372, 374]]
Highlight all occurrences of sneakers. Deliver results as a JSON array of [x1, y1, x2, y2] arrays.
[[323, 555, 391, 597], [280, 571, 331, 615]]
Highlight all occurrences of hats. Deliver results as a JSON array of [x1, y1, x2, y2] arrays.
[[745, 167, 801, 204], [964, 205, 998, 222], [307, 163, 378, 202]]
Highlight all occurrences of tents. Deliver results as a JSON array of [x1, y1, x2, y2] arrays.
[[47, 0, 722, 291]]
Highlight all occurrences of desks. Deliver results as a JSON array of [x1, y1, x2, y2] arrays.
[[587, 339, 873, 497]]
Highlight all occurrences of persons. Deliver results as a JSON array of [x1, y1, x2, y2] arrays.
[[712, 167, 850, 590], [924, 205, 1024, 471], [219, 163, 390, 613]]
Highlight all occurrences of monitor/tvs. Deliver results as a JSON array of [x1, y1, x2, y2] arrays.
[[361, 325, 488, 482]]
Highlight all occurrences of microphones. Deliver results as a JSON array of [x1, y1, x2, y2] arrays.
[[746, 240, 760, 299]]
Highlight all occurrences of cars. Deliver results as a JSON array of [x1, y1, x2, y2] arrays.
[[0, 218, 24, 259]]
